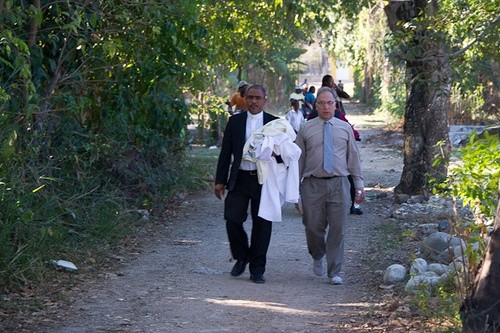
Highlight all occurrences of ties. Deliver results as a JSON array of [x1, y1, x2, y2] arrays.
[[323, 122, 334, 174]]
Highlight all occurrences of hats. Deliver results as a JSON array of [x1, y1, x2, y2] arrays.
[[295, 84, 302, 90], [238, 80, 247, 87]]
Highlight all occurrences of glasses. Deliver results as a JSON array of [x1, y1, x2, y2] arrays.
[[247, 95, 263, 99], [317, 100, 335, 104]]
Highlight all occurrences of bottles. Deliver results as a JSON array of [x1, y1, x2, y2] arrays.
[[354, 190, 362, 208]]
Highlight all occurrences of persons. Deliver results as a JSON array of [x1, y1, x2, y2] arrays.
[[294, 87, 365, 285], [228, 80, 249, 115], [285, 74, 361, 141], [214, 85, 289, 284]]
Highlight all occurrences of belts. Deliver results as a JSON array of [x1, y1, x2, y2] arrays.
[[239, 171, 257, 176]]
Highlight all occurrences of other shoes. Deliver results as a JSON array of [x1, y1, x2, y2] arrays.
[[231, 260, 248, 276], [313, 258, 325, 276], [330, 276, 345, 284], [350, 207, 363, 215], [250, 276, 265, 282]]
